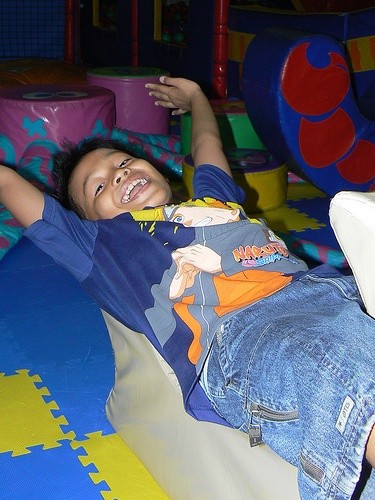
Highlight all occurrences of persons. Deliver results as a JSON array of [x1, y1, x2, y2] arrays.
[[0, 76, 375, 500]]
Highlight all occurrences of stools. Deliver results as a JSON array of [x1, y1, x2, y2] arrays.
[[82, 65, 173, 138], [181, 147, 287, 215], [0, 84, 117, 204], [1, 57, 82, 88], [176, 99, 268, 163]]
